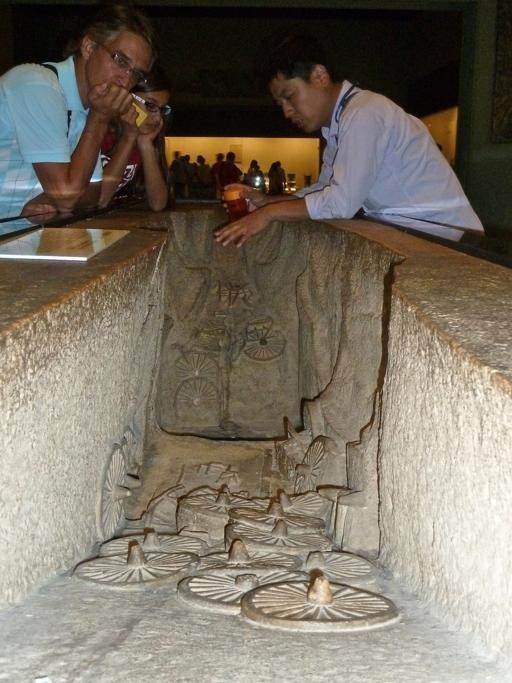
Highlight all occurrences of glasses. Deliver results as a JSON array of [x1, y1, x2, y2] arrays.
[[130, 92, 172, 116], [95, 41, 148, 89]]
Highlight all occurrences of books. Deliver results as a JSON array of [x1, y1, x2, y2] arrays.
[[1, 224, 133, 262]]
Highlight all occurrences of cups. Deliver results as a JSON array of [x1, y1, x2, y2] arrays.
[[224, 188, 247, 221]]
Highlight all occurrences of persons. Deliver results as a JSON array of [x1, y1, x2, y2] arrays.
[[166, 148, 286, 196], [210, 32, 509, 258], [2, 5, 148, 231], [78, 66, 170, 208]]
